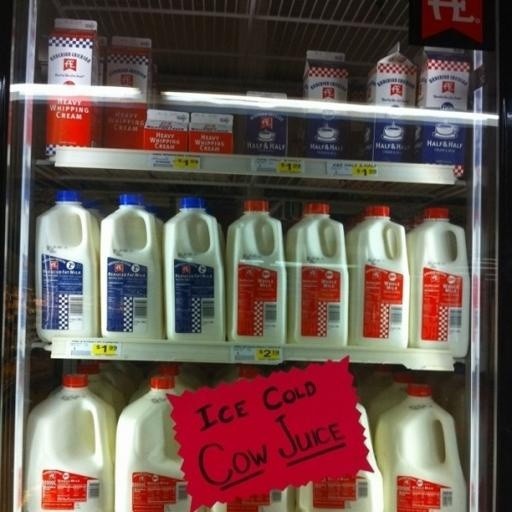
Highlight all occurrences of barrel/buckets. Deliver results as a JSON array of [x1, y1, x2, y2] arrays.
[[286, 202, 348, 350], [98, 193, 162, 340], [372, 374, 469, 512], [347, 206, 408, 348], [35, 191, 98, 338], [408, 206, 472, 358], [116, 372, 191, 512], [24, 376, 114, 510], [162, 196, 224, 341], [225, 200, 287, 343], [296, 403, 383, 509]]
